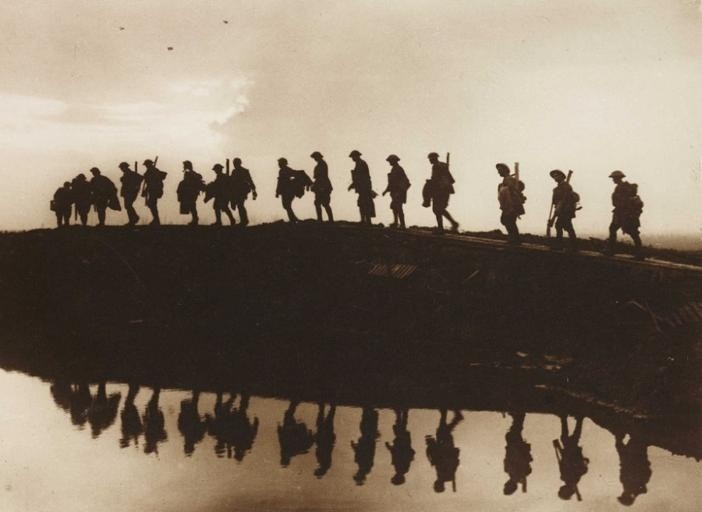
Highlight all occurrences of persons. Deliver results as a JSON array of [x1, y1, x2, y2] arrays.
[[424, 409, 463, 493], [552, 415, 589, 500], [599, 170, 646, 261], [615, 435, 652, 506], [347, 150, 379, 224], [549, 170, 580, 254], [50, 151, 335, 226], [428, 152, 459, 234], [385, 408, 416, 486], [495, 163, 526, 246], [49, 382, 338, 478], [351, 408, 381, 485], [503, 411, 534, 495], [382, 155, 411, 229]]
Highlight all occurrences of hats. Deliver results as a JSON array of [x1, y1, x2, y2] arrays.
[[77, 159, 153, 180], [550, 170, 566, 178], [184, 158, 242, 170], [608, 170, 625, 177], [496, 163, 509, 172], [278, 151, 440, 163]]
